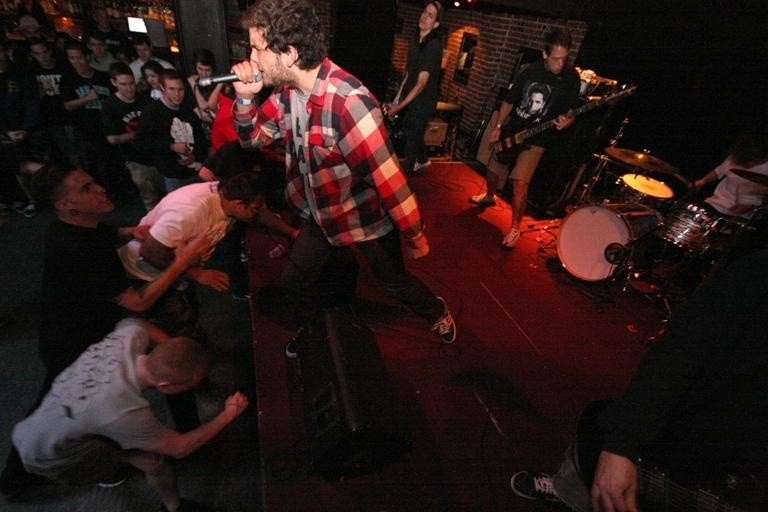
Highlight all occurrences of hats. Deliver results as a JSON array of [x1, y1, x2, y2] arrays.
[[16, 15, 44, 31]]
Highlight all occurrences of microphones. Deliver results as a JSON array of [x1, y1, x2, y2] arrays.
[[198, 71, 262, 87]]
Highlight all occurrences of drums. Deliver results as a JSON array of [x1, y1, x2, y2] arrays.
[[615, 173, 674, 214], [557, 199, 664, 281], [652, 203, 730, 254]]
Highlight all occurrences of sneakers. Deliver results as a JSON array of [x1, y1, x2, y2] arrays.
[[512, 470, 558, 501], [285, 326, 309, 357], [430, 297, 456, 344], [232, 285, 255, 301], [500, 227, 519, 248], [23, 200, 44, 218], [0, 201, 12, 215], [469, 193, 496, 204], [13, 200, 25, 211], [159, 500, 201, 512], [413, 160, 431, 172]]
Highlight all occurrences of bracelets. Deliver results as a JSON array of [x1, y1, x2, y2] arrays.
[[236, 97, 255, 105], [496, 123, 501, 128]]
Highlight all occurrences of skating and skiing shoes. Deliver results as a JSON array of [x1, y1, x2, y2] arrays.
[[96, 471, 124, 489]]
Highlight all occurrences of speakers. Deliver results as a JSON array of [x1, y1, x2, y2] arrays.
[[292, 302, 408, 482]]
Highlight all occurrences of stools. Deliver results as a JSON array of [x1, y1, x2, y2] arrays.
[[433, 100, 464, 160]]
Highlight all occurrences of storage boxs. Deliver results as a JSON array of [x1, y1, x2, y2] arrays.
[[424, 118, 447, 147]]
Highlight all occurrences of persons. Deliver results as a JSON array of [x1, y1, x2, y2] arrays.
[[231, 0, 458, 358], [469, 27, 580, 250], [684, 132, 768, 294], [521, 82, 550, 120], [115, 170, 301, 337], [386, 0, 443, 172], [511, 249, 768, 512], [0, 318, 250, 511], [0, 0, 224, 217], [28, 161, 215, 486]]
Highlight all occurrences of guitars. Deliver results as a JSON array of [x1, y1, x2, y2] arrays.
[[577, 399, 743, 511], [381, 102, 405, 145], [491, 82, 638, 164]]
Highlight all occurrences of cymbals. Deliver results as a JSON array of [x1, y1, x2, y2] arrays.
[[729, 168, 768, 186], [604, 147, 674, 174]]
